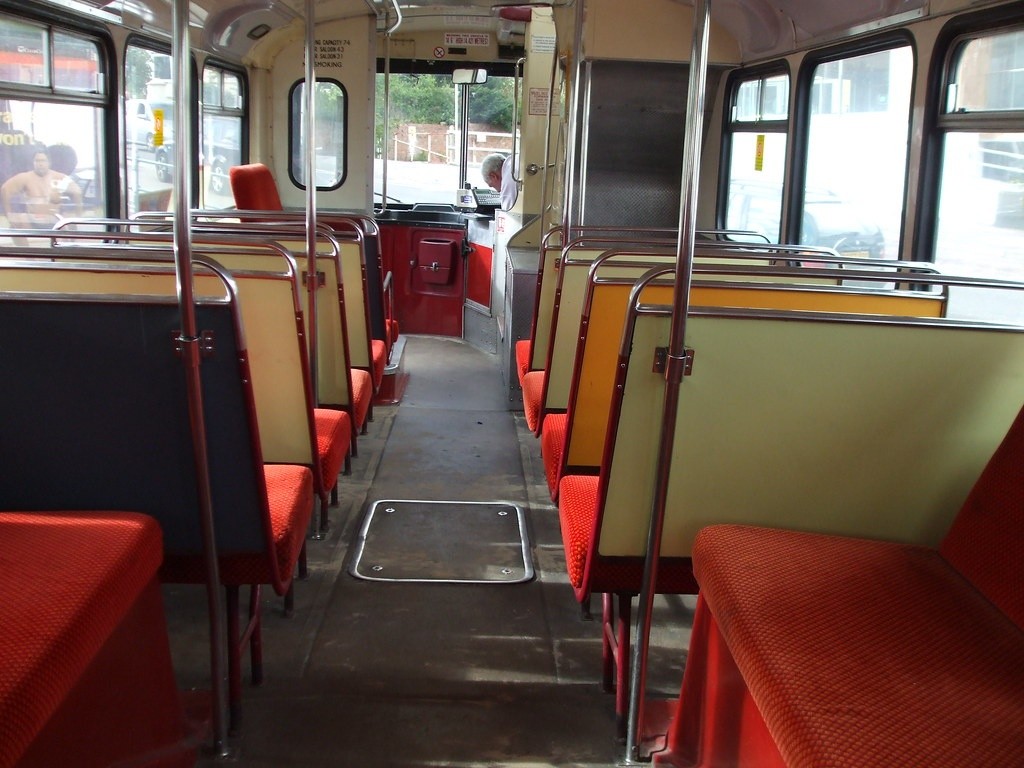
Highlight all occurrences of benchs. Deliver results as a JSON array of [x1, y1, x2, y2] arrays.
[[0, 162, 408, 768], [514, 216, 1024, 767]]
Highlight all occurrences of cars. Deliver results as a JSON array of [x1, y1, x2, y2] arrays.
[[800, 202, 886, 287], [125, 98, 241, 196]]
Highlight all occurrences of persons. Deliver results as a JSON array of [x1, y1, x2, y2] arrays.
[[0, 150, 84, 246], [482, 152, 521, 211]]
